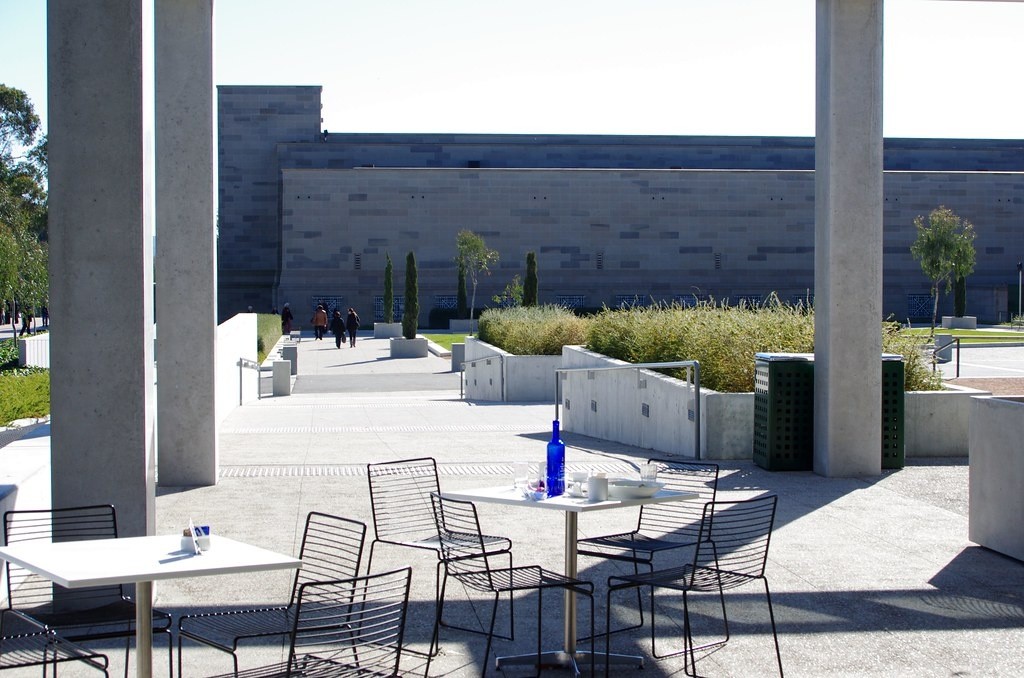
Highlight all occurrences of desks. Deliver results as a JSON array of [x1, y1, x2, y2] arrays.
[[443, 476, 699, 678], [0, 532, 303, 678]]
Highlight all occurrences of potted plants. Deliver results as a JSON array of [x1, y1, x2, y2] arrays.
[[941, 248, 977, 330], [448, 249, 478, 332], [390, 249, 428, 358], [374, 249, 402, 339]]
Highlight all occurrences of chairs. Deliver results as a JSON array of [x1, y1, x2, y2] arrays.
[[356, 456, 513, 656], [576, 458, 730, 658], [423, 492, 595, 678], [607, 493, 779, 678], [178, 511, 367, 678], [205, 565, 412, 678], [0, 608, 109, 678], [3, 504, 174, 678]]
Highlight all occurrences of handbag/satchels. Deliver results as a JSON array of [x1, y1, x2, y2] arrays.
[[342, 332, 346, 344]]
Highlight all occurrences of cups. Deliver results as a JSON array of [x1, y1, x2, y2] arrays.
[[641, 464, 656, 482], [588, 478, 608, 501], [572, 472, 588, 482], [539, 462, 547, 482], [514, 463, 529, 488]]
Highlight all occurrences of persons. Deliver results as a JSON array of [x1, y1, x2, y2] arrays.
[[346, 308, 360, 348], [20, 306, 32, 336], [41, 297, 49, 327], [282, 303, 293, 334], [312, 298, 329, 340], [246, 306, 254, 313], [271, 307, 279, 314], [331, 310, 346, 349], [1, 297, 19, 325]]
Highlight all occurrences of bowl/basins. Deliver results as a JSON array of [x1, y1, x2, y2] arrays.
[[525, 492, 543, 500], [608, 481, 665, 499]]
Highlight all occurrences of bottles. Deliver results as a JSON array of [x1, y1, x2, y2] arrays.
[[547, 421, 565, 498]]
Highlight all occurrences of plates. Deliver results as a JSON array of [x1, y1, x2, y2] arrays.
[[566, 489, 588, 497]]
